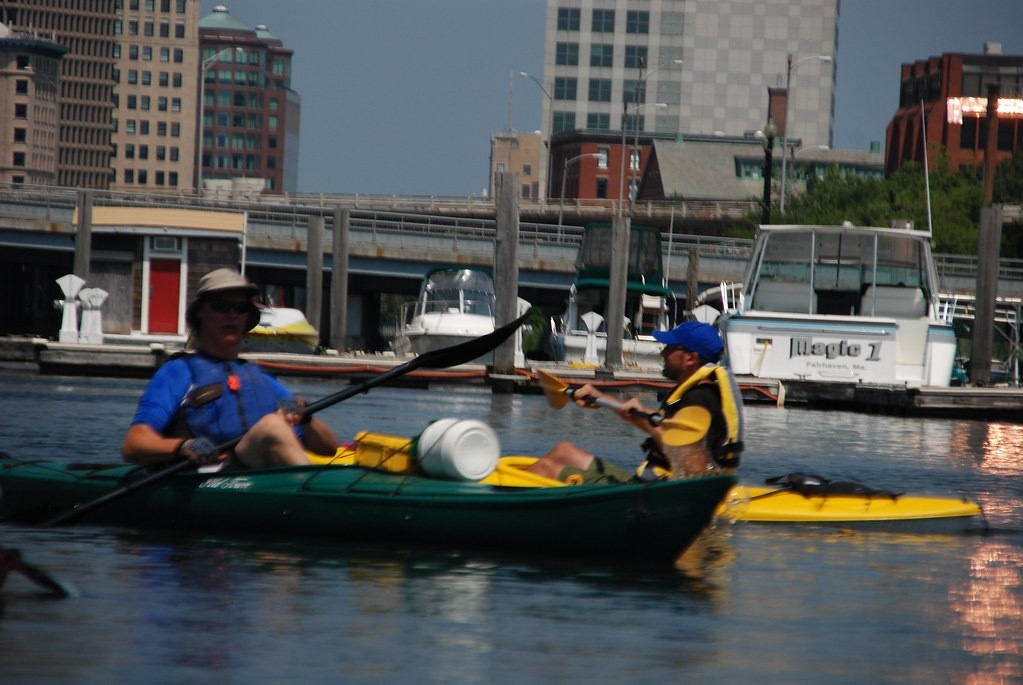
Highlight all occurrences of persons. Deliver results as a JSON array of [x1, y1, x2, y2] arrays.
[[122, 269, 337, 466], [520, 321, 738, 485]]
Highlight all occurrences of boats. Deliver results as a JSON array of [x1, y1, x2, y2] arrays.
[[549, 222, 678, 366], [717, 220, 957, 387], [304, 431, 981, 523], [392, 266, 495, 364], [965, 359, 1009, 383], [953, 357, 970, 382], [0, 451, 739, 568], [241, 307, 319, 354]]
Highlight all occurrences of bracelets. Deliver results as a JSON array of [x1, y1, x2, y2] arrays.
[[173, 436, 191, 460]]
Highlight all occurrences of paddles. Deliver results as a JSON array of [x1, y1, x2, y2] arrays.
[[535, 368, 714, 448], [50, 304, 536, 527]]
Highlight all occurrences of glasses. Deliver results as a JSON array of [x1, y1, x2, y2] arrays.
[[210, 298, 251, 315], [665, 344, 686, 354]]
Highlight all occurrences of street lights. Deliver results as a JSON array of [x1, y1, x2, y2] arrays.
[[788, 145, 830, 198], [780, 56, 832, 212], [762, 115, 776, 224], [632, 57, 682, 200], [556, 153, 602, 243], [197, 47, 243, 205], [619, 101, 667, 215], [520, 72, 553, 198]]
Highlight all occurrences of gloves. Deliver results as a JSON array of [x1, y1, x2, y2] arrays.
[[174, 437, 217, 466]]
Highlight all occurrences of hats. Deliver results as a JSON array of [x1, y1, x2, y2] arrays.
[[185, 268, 261, 335], [653, 321, 724, 361]]
